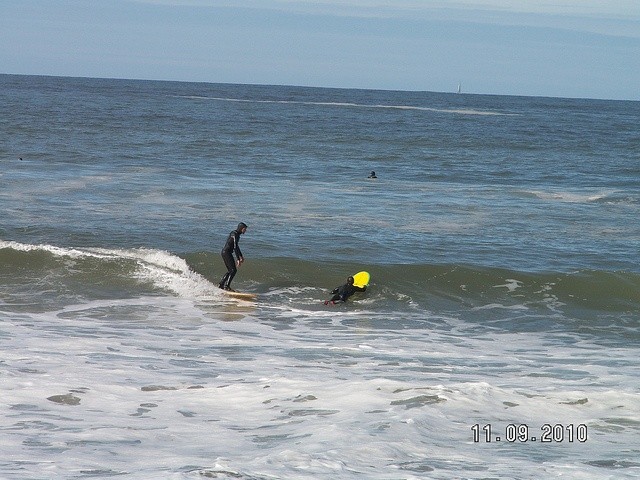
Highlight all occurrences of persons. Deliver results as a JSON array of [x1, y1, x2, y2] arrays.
[[218, 222, 248, 292], [368, 170, 377, 179], [323, 276, 367, 305]]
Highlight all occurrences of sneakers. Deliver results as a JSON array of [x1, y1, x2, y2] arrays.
[[331, 301, 334, 304], [324, 301, 328, 305], [219, 284, 235, 291]]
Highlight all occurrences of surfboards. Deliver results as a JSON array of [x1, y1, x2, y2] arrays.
[[352, 271, 370, 288], [219, 288, 255, 297]]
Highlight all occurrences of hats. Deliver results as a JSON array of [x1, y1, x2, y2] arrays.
[[238, 222, 247, 234], [348, 277, 354, 284]]
